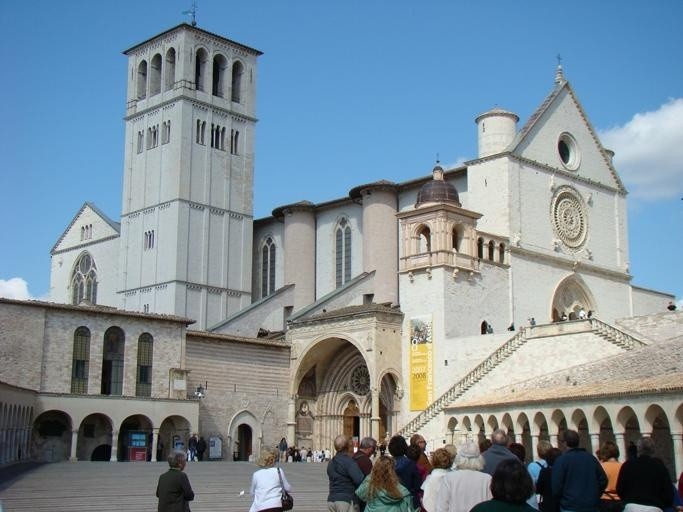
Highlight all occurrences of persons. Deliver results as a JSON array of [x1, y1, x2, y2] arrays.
[[469, 459, 540, 512], [481, 429, 521, 476], [197, 437, 207, 461], [410, 434, 434, 484], [355, 455, 414, 512], [247, 447, 293, 512], [388, 435, 423, 511], [434, 440, 493, 512], [443, 444, 457, 472], [615, 436, 675, 512], [327, 434, 365, 512], [596, 440, 624, 512], [352, 438, 377, 511], [527, 440, 553, 510], [274, 437, 325, 463], [509, 443, 525, 463], [156, 450, 195, 512], [188, 433, 198, 461], [551, 430, 609, 512], [420, 447, 454, 511], [535, 448, 562, 512], [478, 439, 492, 455], [405, 443, 429, 512]]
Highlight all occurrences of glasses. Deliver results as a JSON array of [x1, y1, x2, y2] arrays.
[[369, 444, 376, 448]]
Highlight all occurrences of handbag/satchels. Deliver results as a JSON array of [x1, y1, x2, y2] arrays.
[[277, 467, 294, 510]]
